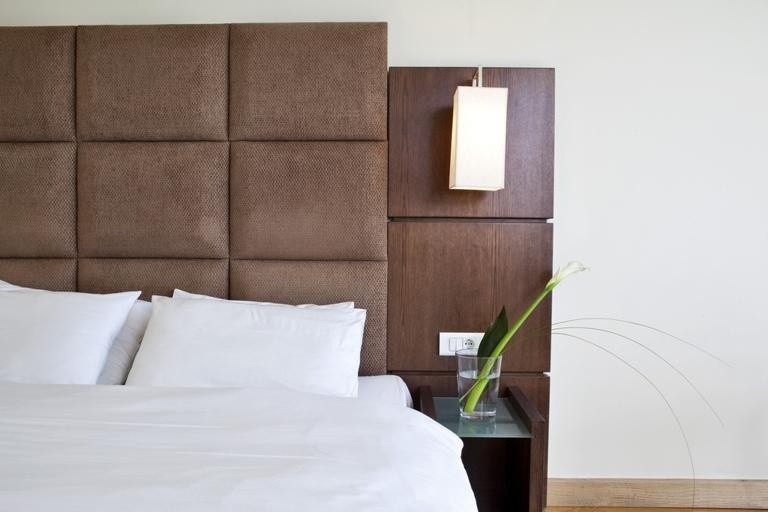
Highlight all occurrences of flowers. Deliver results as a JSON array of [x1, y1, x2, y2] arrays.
[[461, 257, 588, 419]]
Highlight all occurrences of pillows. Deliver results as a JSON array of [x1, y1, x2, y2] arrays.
[[1, 281, 142, 386], [173, 287, 356, 312], [94, 295, 155, 386], [121, 294, 365, 399]]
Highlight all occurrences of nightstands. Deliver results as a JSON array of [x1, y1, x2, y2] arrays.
[[417, 384, 547, 512]]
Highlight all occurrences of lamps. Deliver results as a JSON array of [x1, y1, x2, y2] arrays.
[[447, 66, 509, 193]]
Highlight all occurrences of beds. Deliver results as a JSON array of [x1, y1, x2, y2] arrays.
[[0, 23, 478, 512]]
